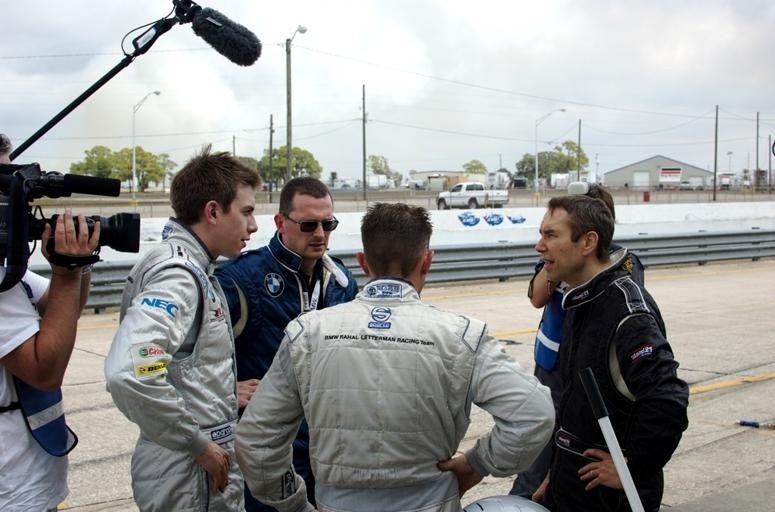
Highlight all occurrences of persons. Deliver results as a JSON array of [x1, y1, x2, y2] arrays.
[[214, 177, 357, 511], [105, 150, 263, 511], [233, 200, 556, 512], [1, 134, 101, 511], [511, 183, 686, 512]]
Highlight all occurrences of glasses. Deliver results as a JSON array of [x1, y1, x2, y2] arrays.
[[284, 213, 339, 233]]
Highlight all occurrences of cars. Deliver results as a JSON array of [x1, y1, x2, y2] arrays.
[[680, 177, 730, 191]]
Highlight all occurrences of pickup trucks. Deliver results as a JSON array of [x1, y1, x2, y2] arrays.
[[436, 183, 509, 210]]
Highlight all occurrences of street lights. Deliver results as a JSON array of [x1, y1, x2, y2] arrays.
[[535, 109, 566, 193], [132, 92, 161, 200], [286, 24, 308, 181]]
